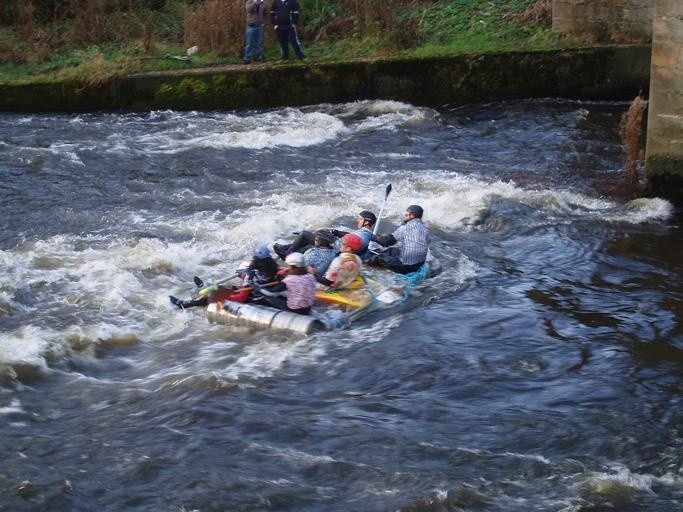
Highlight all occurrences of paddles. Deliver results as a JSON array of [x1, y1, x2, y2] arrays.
[[131, 55, 192, 62], [191, 274, 239, 301], [324, 284, 436, 290], [365, 185, 392, 259], [212, 282, 278, 300]]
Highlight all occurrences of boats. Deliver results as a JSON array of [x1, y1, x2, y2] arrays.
[[207, 245, 441, 335]]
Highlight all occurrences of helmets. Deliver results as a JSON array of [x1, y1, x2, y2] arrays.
[[316, 230, 360, 251], [254, 246, 270, 259], [286, 252, 304, 267], [360, 211, 375, 226], [407, 205, 423, 218]]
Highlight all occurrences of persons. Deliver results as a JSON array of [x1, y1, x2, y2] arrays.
[[243, 0, 268, 64], [270, 0, 309, 64], [168, 204, 431, 315]]
